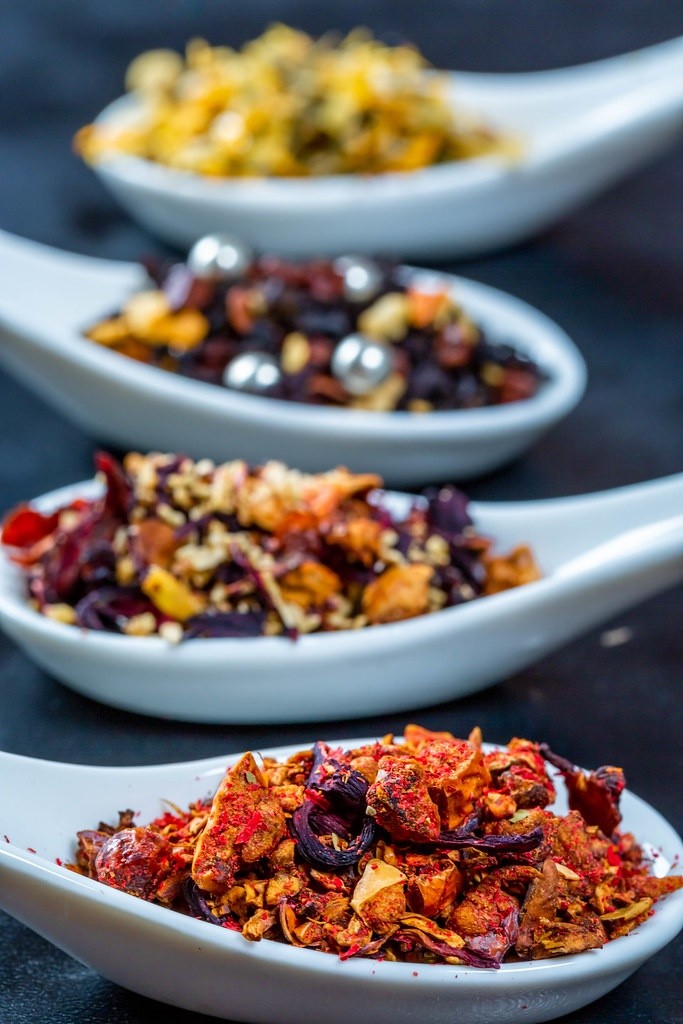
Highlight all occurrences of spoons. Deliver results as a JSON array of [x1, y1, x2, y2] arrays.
[[0, 471, 683, 719], [81, 37, 683, 254], [0, 231, 586, 479], [0, 737, 683, 1024]]
